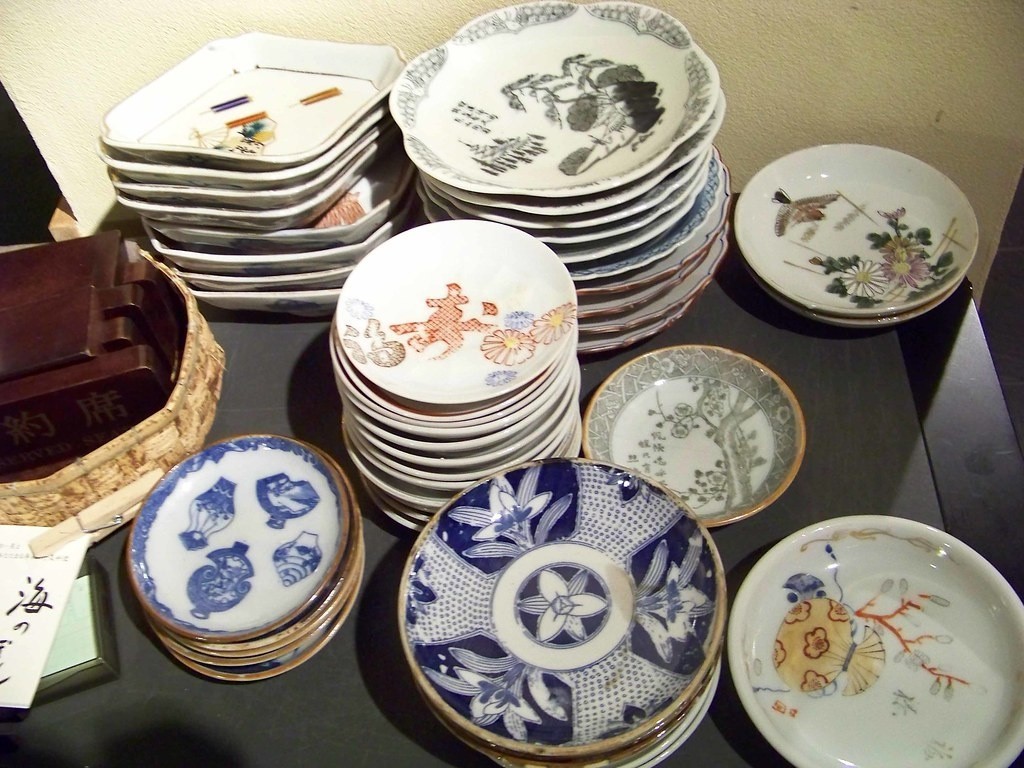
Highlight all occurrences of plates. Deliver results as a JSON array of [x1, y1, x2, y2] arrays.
[[739, 142, 979, 327], [389, 2, 731, 354], [728, 515, 1024, 768], [330, 218, 584, 531], [127, 431, 364, 683], [99, 31, 413, 317], [396, 458, 726, 767], [582, 343, 808, 528]]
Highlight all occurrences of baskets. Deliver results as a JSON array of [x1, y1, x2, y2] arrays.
[[1, 241, 226, 538]]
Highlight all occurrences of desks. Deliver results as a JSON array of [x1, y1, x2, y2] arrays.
[[0, 215, 1024, 767]]
